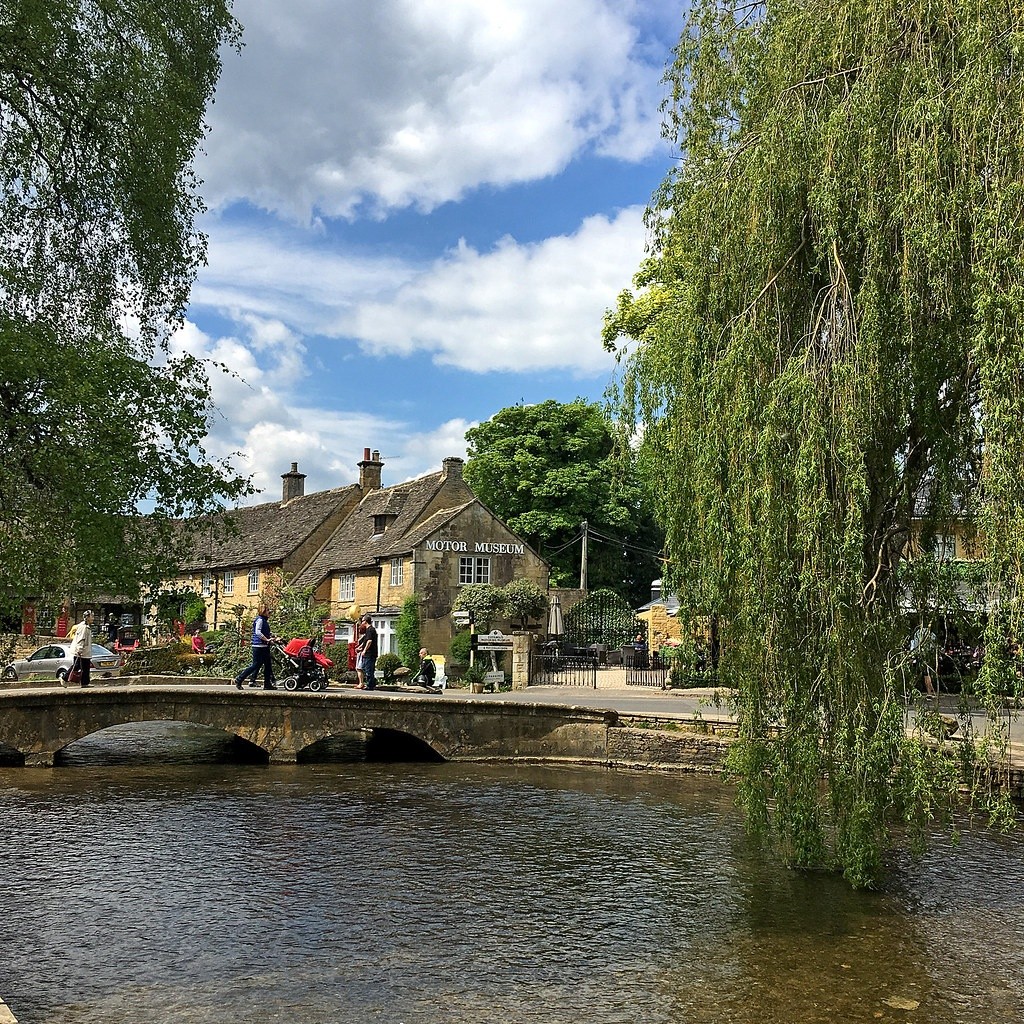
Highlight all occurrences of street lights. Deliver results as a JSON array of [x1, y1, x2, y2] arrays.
[[373, 556, 382, 612], [212, 567, 219, 630]]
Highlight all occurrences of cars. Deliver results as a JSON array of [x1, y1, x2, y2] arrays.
[[4, 643, 125, 681]]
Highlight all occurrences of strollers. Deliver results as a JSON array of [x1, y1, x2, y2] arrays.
[[198, 645, 218, 654], [271, 638, 333, 691]]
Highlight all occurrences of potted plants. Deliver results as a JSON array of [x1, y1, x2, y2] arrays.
[[463, 657, 493, 693]]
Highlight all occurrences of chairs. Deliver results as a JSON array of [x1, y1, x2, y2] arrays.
[[570, 643, 664, 670]]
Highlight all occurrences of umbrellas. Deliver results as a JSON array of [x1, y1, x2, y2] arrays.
[[548, 595, 564, 642]]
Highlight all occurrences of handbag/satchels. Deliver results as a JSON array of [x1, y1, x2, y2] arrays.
[[68, 653, 83, 683]]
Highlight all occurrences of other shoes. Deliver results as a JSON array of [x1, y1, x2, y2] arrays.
[[81, 684, 95, 688], [354, 685, 363, 689], [263, 687, 277, 690], [249, 681, 261, 687], [59, 677, 67, 688], [363, 685, 373, 690], [235, 680, 243, 690]]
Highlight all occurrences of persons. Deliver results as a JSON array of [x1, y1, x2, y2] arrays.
[[233, 606, 278, 690], [192, 629, 205, 654], [59, 610, 95, 688], [354, 615, 378, 690], [409, 648, 436, 686]]
